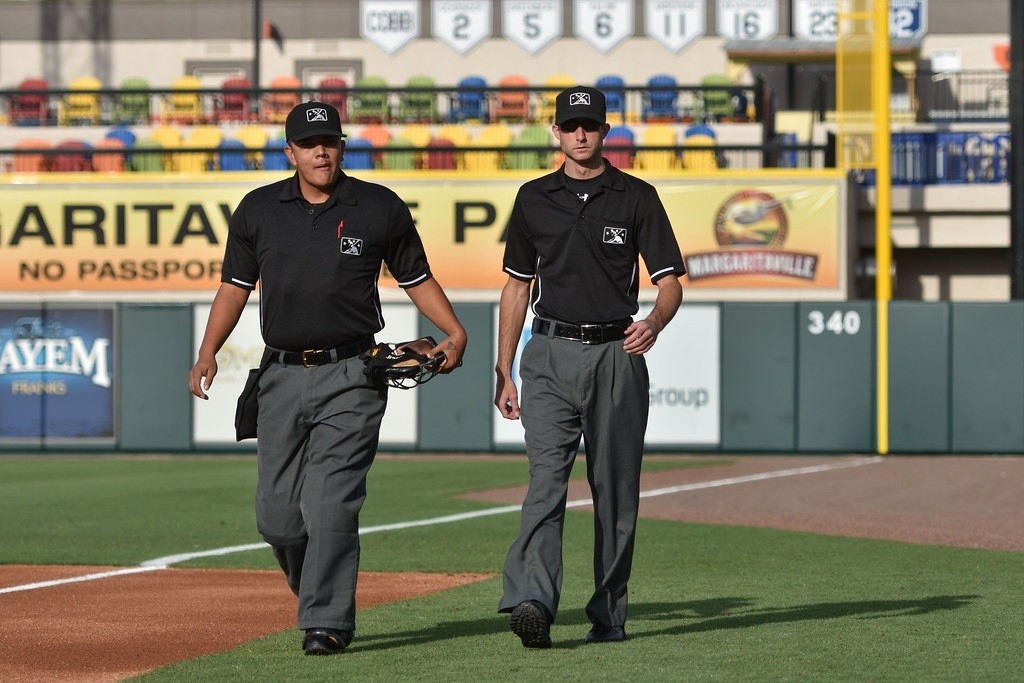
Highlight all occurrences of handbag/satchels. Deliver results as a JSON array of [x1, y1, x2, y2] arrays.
[[234, 348, 274, 442]]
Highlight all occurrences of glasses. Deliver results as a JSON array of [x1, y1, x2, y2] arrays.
[[555, 118, 604, 132]]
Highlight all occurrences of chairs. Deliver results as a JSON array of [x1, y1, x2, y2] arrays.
[[1, 75, 734, 172]]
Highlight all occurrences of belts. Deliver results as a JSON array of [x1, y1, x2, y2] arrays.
[[267, 333, 375, 368], [532, 316, 633, 345]]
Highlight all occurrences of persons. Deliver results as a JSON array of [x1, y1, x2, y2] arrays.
[[494, 86, 687, 648], [189, 101, 468, 655]]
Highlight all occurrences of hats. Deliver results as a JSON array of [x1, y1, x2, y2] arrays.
[[556, 86, 606, 125], [286, 101, 347, 143]]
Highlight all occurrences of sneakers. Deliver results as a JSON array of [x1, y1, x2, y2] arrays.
[[302, 627, 355, 655], [585, 624, 626, 642], [510, 599, 553, 648]]
